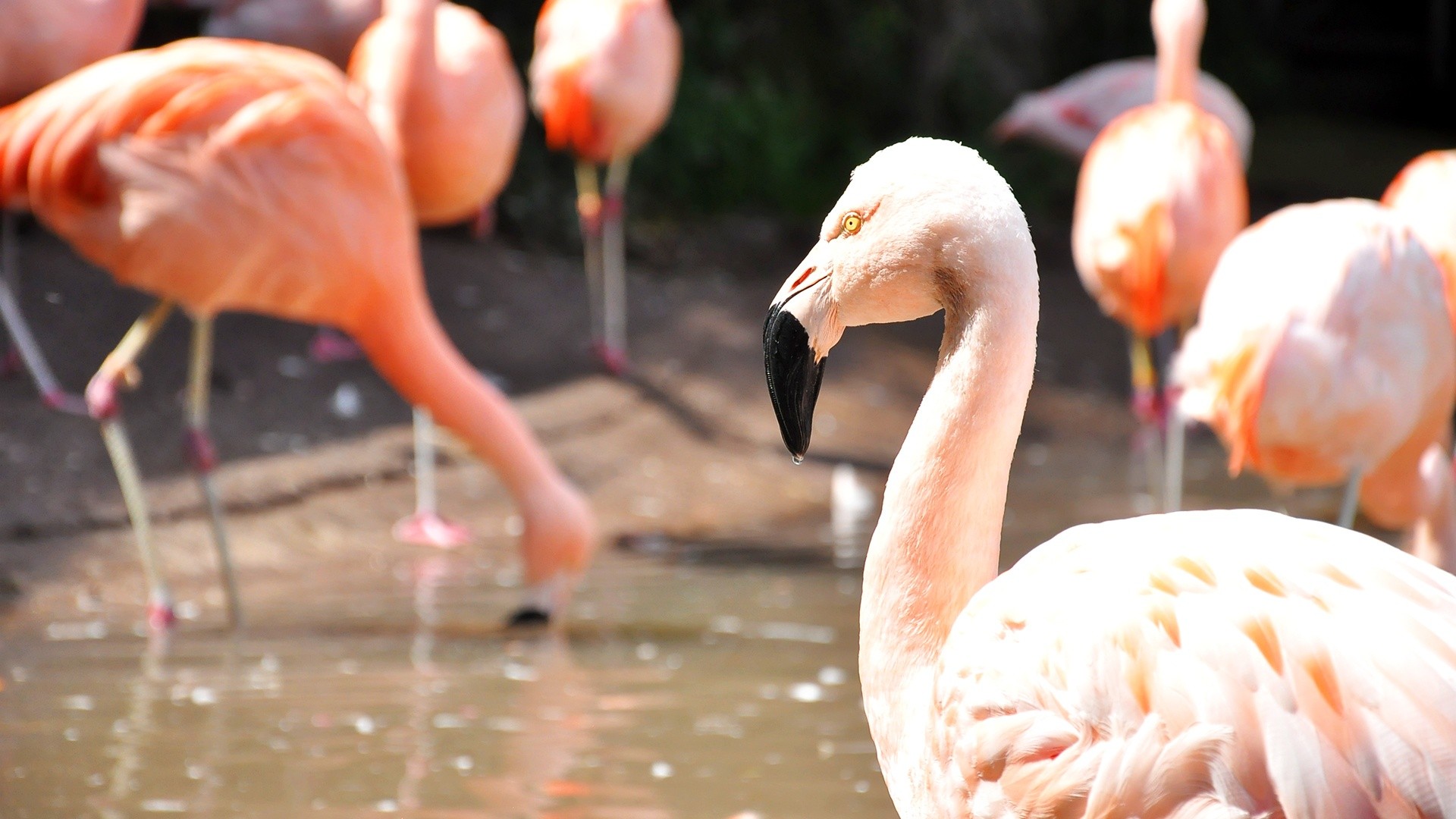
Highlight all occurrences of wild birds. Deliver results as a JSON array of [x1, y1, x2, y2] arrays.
[[0, 0, 681, 665], [760, 0, 1456, 819]]
